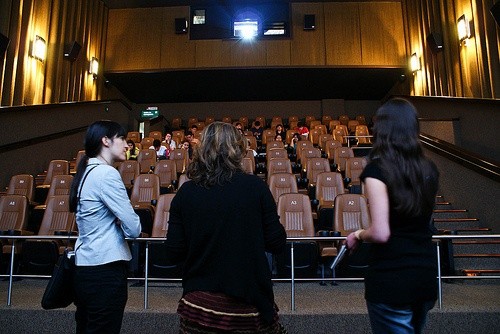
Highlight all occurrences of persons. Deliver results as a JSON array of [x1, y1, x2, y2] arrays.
[[165, 123, 288, 334], [118, 107, 310, 161], [341, 93, 439, 334], [72, 118, 141, 334]]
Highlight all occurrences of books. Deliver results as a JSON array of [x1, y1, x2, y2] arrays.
[[330, 244, 346, 269]]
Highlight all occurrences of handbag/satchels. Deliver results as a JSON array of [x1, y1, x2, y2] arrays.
[[41, 249, 76, 310]]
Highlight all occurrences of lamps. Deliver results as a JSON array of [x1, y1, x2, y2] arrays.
[[31, 34, 46, 63], [408, 53, 422, 75], [88, 57, 99, 80], [456, 14, 469, 47]]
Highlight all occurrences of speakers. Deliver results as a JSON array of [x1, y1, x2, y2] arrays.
[[174, 17, 188, 35], [303, 14, 317, 30], [426, 31, 442, 54], [67, 40, 83, 61]]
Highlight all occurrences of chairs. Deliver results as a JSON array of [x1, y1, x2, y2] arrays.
[[0, 113, 378, 286]]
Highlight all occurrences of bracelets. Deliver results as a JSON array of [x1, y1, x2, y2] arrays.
[[354, 228, 364, 241]]
